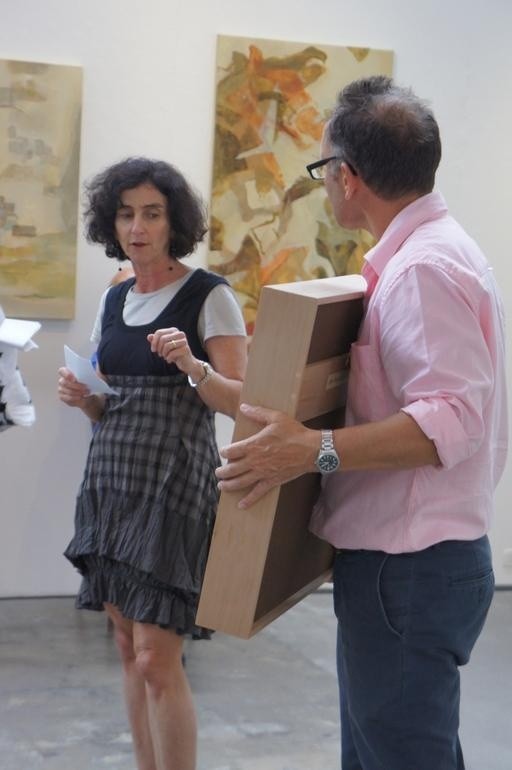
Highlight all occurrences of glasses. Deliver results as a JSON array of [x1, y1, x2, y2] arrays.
[[303, 154, 361, 188]]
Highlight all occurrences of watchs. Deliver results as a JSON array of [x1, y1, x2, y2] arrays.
[[185, 362, 214, 390], [315, 428, 340, 477]]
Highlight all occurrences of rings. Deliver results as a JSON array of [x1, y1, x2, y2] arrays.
[[171, 340, 180, 347]]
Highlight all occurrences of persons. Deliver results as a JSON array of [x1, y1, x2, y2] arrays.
[[215, 74, 511, 770], [58, 156, 248, 770]]
